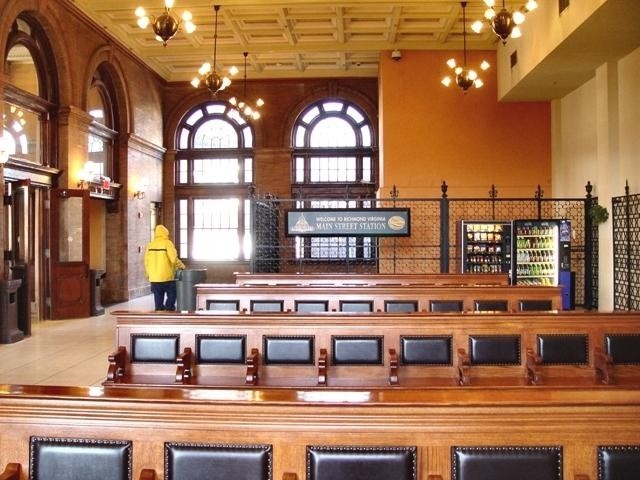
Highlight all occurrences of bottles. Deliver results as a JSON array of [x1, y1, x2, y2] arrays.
[[468, 254, 501, 273], [517, 226, 556, 287]]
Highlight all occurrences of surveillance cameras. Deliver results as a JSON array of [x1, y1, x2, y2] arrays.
[[391, 51, 401, 61]]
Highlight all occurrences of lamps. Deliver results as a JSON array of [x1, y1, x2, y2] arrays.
[[229, 51, 266, 124], [133, 0, 198, 50], [440, 1, 492, 98], [472, 0, 538, 43], [189, 4, 239, 98]]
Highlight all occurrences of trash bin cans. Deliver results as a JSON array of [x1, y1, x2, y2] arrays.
[[90, 269, 105, 316], [2, 278, 25, 344], [174, 268, 208, 311]]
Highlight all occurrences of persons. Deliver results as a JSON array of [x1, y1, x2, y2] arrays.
[[144, 225, 187, 310]]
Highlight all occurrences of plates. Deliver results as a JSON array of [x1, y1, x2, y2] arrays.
[[388, 216, 406, 230]]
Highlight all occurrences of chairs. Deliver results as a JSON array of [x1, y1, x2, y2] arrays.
[[0, 270, 639, 478]]
